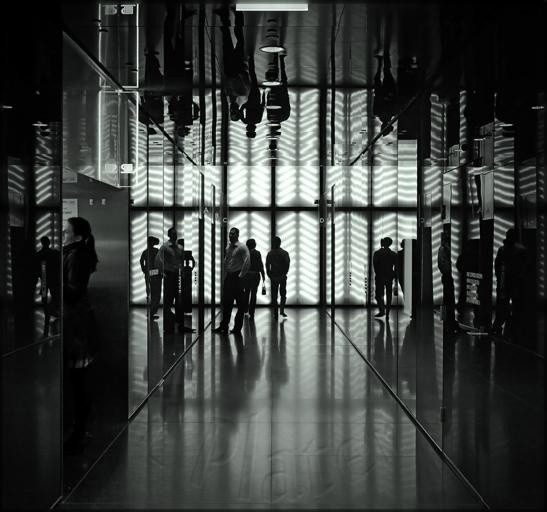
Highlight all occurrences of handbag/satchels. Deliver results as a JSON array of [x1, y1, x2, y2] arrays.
[[393, 279, 399, 297], [262, 284, 266, 295]]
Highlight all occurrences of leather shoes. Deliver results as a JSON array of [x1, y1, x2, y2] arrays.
[[212, 327, 228, 335], [280, 312, 287, 317], [230, 327, 241, 333], [374, 313, 386, 317]]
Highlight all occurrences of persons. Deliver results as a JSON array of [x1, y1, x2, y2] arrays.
[[266, 236, 289, 321], [244, 239, 264, 320], [36, 236, 59, 321], [140, 227, 196, 337], [46, 216, 97, 456], [211, 228, 252, 334], [373, 237, 403, 322], [490, 226, 530, 345]]
